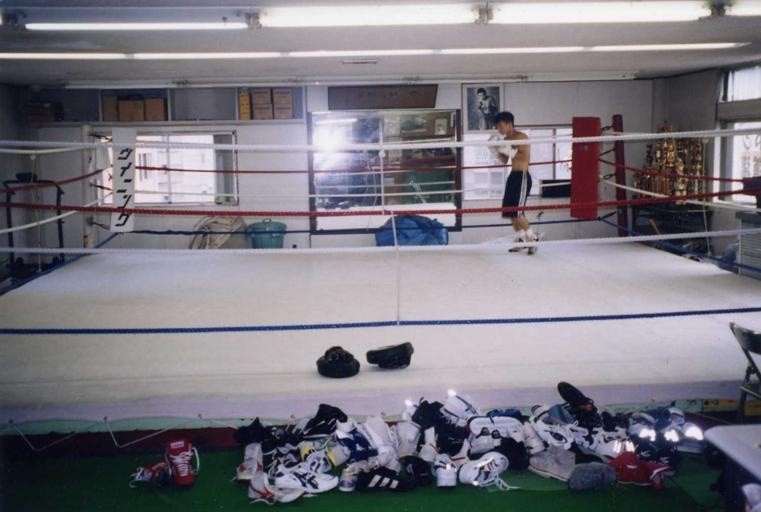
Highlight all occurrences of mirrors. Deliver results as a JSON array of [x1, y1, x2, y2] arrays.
[[306, 109, 462, 235]]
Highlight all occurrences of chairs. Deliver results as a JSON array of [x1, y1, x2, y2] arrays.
[[729, 322, 761, 415]]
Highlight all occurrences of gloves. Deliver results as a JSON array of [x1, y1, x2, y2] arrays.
[[487, 134, 519, 165]]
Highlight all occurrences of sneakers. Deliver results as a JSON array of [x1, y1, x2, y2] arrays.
[[508, 237, 540, 255], [129, 433, 200, 488]]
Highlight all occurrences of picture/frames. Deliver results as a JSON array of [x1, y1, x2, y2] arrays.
[[461, 83, 504, 134], [326, 83, 438, 110]]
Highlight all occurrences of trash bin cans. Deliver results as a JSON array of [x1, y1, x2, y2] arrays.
[[247, 218, 287, 249]]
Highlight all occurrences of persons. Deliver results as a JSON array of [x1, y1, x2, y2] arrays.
[[486, 111, 540, 255], [476, 88, 498, 130]]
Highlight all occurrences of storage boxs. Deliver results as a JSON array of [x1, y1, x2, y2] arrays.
[[102, 92, 165, 121], [250, 88, 292, 119]]
